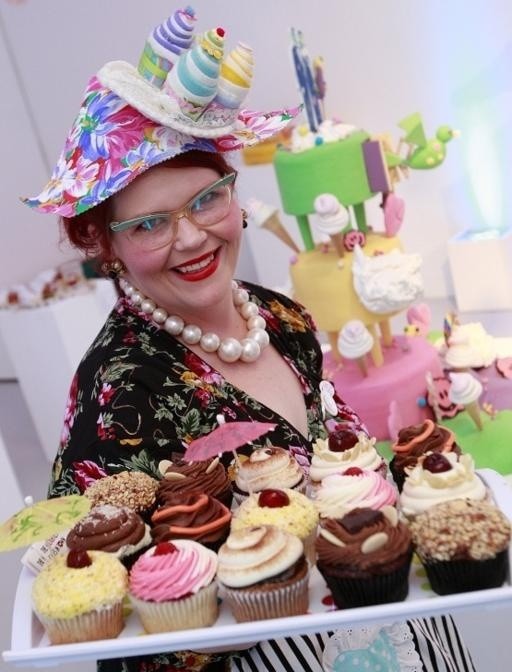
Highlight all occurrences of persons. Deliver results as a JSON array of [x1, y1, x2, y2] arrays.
[[17, 2, 477, 672]]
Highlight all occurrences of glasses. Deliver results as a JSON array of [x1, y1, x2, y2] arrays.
[[109, 170, 240, 258]]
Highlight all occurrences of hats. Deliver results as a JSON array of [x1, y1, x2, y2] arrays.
[[21, 62, 303, 222]]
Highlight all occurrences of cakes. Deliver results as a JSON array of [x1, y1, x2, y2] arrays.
[[243, 25, 464, 440]]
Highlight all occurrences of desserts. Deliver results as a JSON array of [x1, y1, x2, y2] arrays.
[[29, 419, 512, 648]]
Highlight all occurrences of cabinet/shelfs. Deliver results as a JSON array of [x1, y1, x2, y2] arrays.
[[0, 276, 119, 469]]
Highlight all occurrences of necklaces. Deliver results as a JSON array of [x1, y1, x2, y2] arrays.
[[115, 279, 270, 363]]
